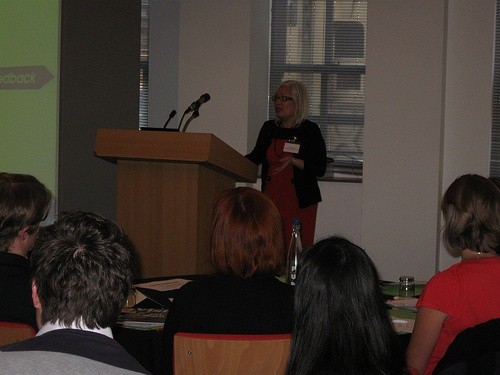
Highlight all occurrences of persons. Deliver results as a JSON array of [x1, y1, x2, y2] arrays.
[[285, 235, 412, 374], [1, 172, 55, 330], [1, 209, 152, 374], [405, 174, 500, 374], [240, 79, 328, 274], [152, 185, 297, 375]]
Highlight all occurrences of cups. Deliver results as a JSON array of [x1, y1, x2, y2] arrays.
[[121, 287, 136, 314], [399, 275, 415, 297]]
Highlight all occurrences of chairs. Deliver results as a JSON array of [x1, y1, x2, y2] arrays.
[[172, 331, 293, 374]]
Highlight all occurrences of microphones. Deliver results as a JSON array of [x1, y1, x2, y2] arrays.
[[186, 93, 211, 114], [161, 110, 176, 129], [182, 111, 199, 131]]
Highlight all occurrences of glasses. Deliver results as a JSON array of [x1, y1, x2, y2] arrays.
[[272, 95, 294, 102]]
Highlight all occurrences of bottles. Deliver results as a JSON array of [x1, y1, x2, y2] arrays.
[[286, 217, 303, 286]]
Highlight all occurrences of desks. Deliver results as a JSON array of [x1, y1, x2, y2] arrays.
[[115, 272, 426, 336]]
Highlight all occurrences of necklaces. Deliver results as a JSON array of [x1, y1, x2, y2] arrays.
[[274, 121, 294, 158], [461, 246, 491, 257]]
